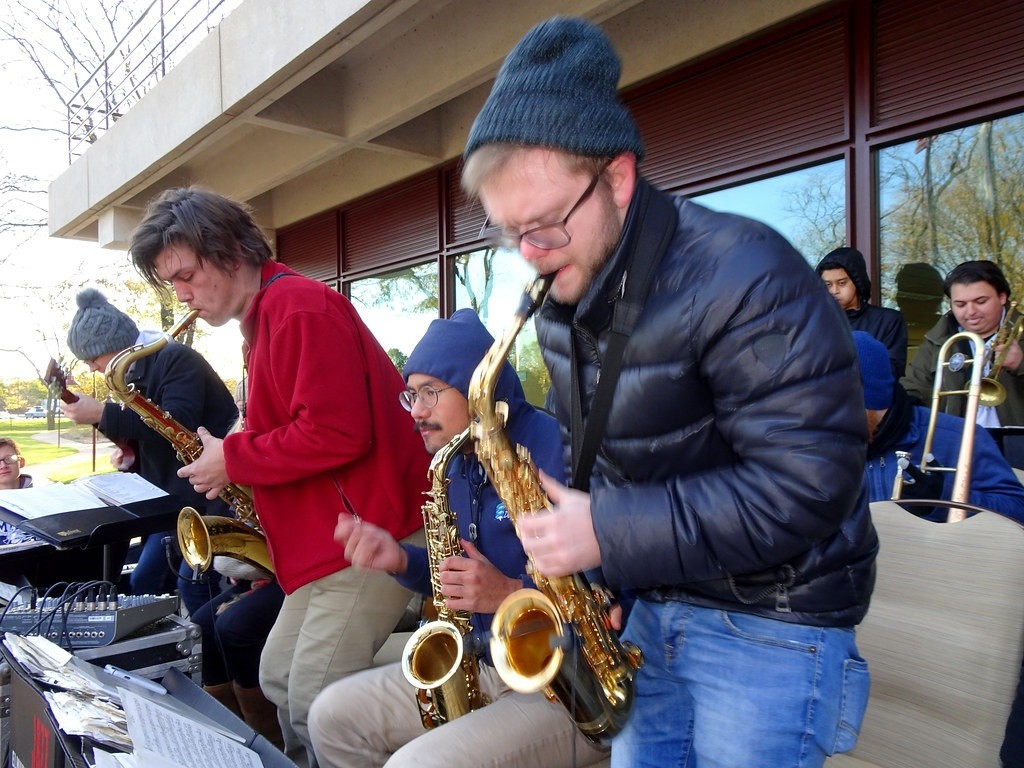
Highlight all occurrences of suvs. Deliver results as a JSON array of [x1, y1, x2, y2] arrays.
[[24, 406, 48, 418]]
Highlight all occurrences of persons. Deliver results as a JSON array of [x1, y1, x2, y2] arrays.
[[0, 437, 33, 488], [896, 260, 946, 345], [310, 308, 616, 768], [460, 15, 882, 768], [54, 288, 239, 613], [814, 243, 1024, 768], [126, 185, 431, 768]]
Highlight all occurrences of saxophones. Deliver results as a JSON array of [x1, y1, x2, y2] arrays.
[[466, 268, 644, 742], [400, 424, 480, 734], [103, 310, 277, 583]]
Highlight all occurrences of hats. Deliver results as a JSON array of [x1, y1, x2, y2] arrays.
[[462, 12, 645, 158], [851, 331, 895, 412], [67, 289, 140, 361]]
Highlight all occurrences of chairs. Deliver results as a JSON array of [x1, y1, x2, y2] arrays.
[[811, 493, 1024, 768]]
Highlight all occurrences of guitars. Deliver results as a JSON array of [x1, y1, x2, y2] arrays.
[[43, 357, 130, 463]]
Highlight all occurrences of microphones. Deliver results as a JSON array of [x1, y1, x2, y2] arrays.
[[562, 624, 575, 651], [161, 535, 176, 545]]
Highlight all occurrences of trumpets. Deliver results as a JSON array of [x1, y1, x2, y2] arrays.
[[964, 295, 1023, 407]]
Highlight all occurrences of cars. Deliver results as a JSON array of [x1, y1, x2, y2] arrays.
[[51, 407, 64, 415]]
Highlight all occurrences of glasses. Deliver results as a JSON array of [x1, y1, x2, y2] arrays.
[[83, 357, 100, 367], [477, 157, 609, 259], [399, 386, 455, 412], [0, 454, 21, 464]]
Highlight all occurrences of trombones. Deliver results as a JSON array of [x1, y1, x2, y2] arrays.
[[888, 329, 988, 528]]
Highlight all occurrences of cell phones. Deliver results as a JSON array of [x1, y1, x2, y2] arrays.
[[106, 665, 167, 696]]
